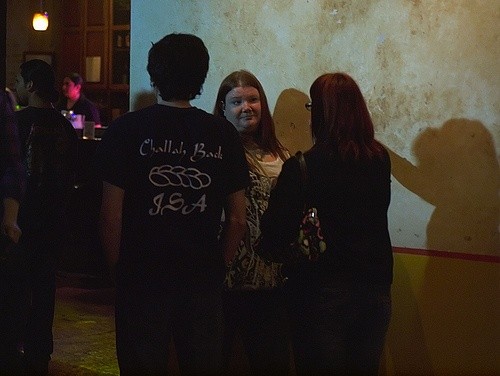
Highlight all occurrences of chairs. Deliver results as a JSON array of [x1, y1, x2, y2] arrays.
[[18, 125, 80, 269]]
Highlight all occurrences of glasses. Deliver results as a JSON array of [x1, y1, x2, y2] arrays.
[[305, 102, 313, 111]]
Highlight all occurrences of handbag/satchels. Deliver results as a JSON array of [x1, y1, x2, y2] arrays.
[[252, 151, 328, 264]]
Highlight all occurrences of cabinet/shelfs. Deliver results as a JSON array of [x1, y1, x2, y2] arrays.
[[58, 0, 130, 128]]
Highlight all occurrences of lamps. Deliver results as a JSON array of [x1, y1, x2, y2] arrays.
[[31, 0, 50, 32]]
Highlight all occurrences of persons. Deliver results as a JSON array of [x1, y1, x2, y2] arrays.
[[0, 59, 78, 376], [90, 33, 252, 376], [55, 72, 100, 125], [259, 73, 394, 376], [213, 70, 303, 376]]
